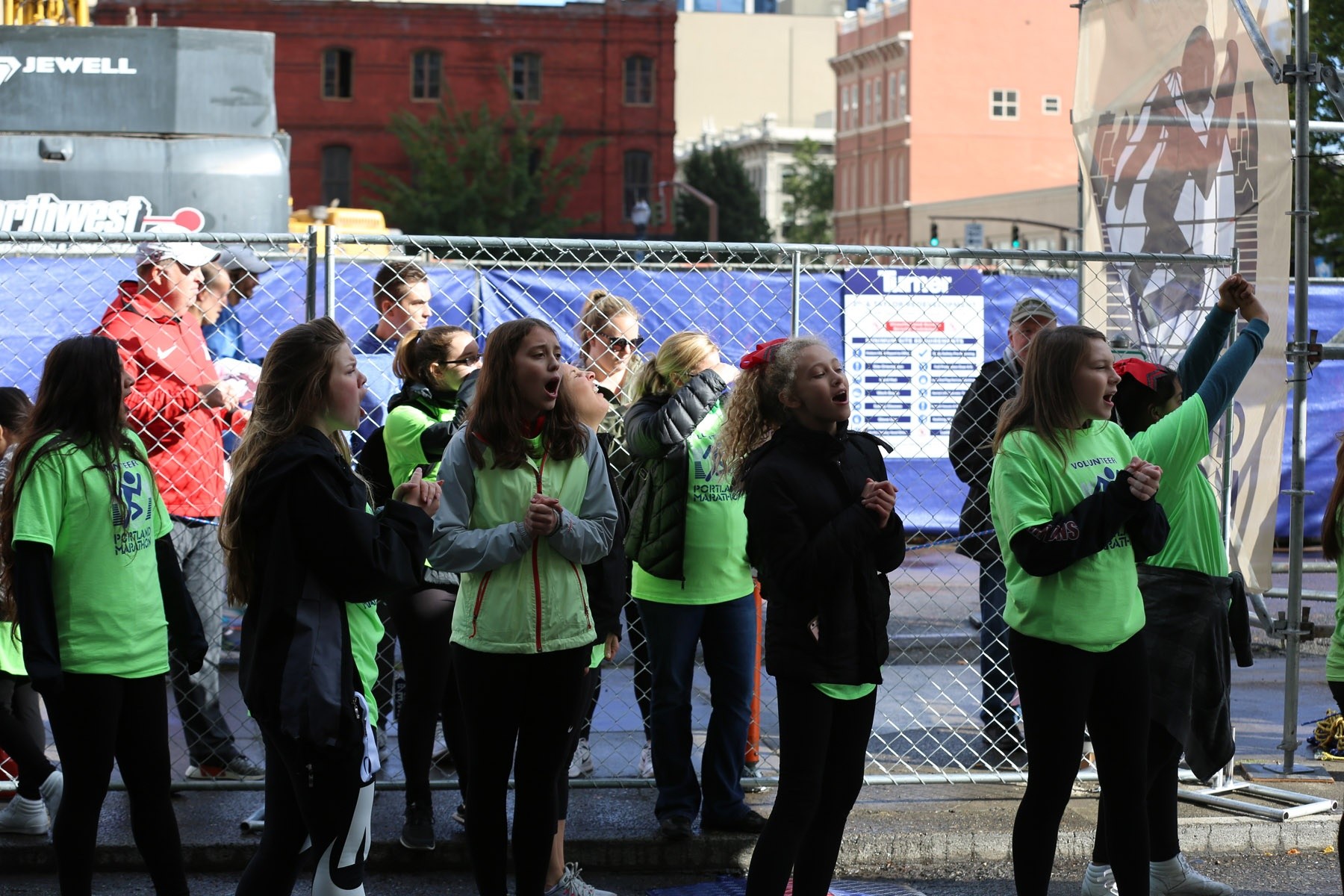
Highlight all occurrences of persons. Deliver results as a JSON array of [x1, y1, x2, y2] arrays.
[[710, 337, 907, 896], [948, 298, 1091, 771], [989, 325, 1170, 896], [1081, 273, 1270, 896], [1321, 440, 1344, 893], [0, 220, 770, 851], [216, 316, 445, 896], [0, 333, 210, 896], [425, 318, 618, 896]]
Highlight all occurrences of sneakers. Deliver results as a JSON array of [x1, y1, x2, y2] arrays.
[[452, 803, 465, 824], [0, 794, 49, 835], [639, 743, 655, 777], [39, 771, 64, 832], [517, 862, 617, 896], [432, 722, 448, 761], [1150, 853, 1233, 896], [399, 793, 436, 850], [377, 728, 388, 763], [185, 754, 265, 781], [1081, 862, 1119, 896], [568, 738, 593, 777]]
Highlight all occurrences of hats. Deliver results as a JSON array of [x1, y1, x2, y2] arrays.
[[135, 223, 221, 268], [1009, 297, 1056, 326], [217, 246, 274, 274]]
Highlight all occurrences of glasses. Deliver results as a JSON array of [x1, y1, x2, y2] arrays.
[[595, 332, 644, 352], [435, 353, 480, 366]]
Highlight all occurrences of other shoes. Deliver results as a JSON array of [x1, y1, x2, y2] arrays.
[[660, 816, 694, 841], [982, 718, 1025, 749], [700, 807, 768, 834], [742, 762, 767, 793]]
